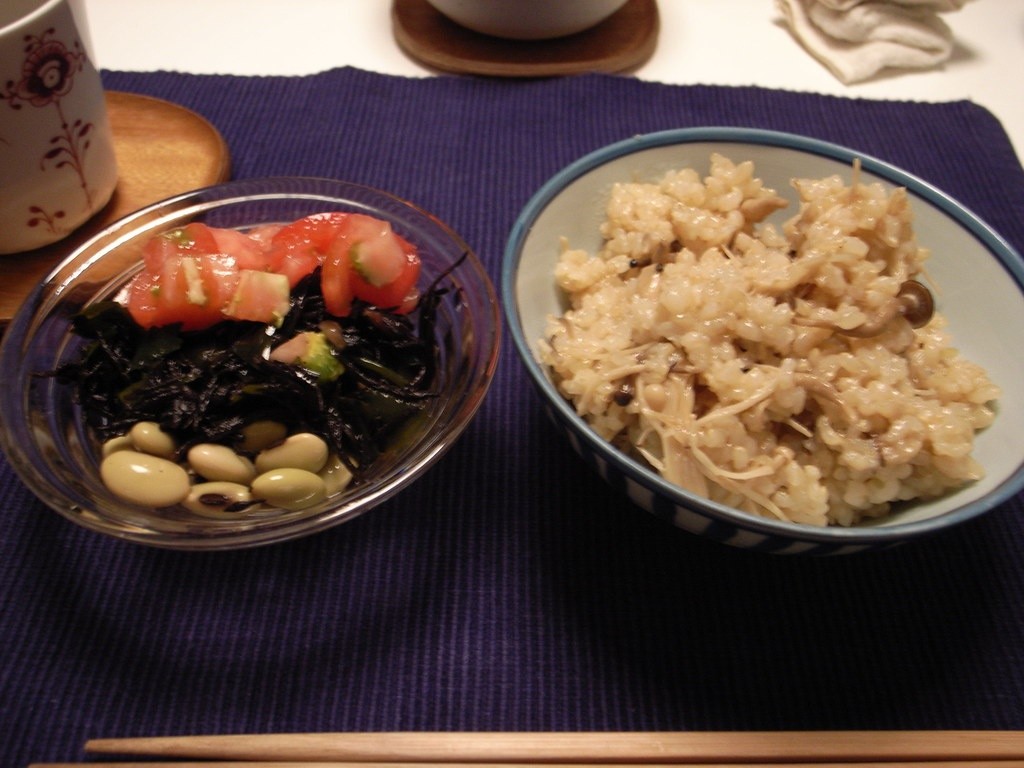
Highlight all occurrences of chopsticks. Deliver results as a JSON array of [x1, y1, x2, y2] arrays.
[[21, 728, 1024, 768]]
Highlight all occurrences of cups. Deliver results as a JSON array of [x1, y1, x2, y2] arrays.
[[0, 0, 122, 257]]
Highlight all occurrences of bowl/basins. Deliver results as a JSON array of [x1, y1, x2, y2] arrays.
[[498, 123, 1023, 556], [0, 176, 503, 551], [422, 0, 634, 40]]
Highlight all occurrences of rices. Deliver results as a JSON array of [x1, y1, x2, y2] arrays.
[[536, 153, 1000, 525]]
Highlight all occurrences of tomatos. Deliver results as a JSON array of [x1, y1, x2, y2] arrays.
[[125, 208, 422, 378]]
